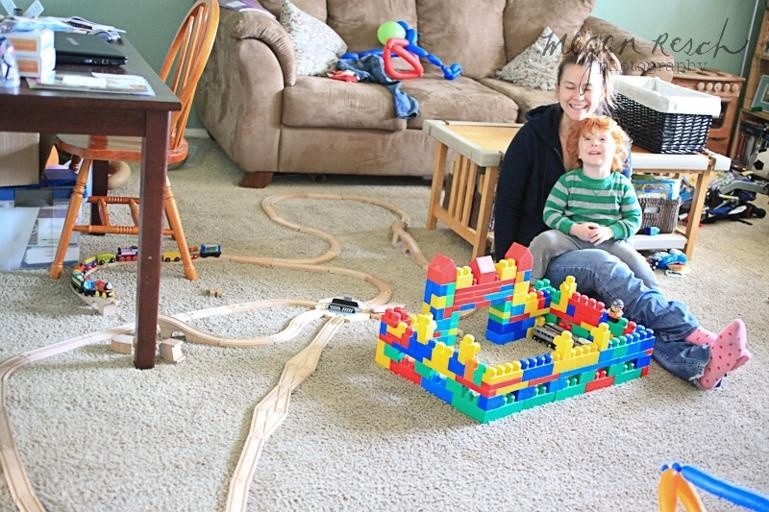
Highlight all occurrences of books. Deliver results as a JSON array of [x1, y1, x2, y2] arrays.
[[218, 0, 276, 19], [736, 119, 769, 164], [25, 69, 159, 95]]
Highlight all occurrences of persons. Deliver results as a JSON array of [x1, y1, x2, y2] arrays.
[[523, 113, 662, 292], [494, 30, 753, 392]]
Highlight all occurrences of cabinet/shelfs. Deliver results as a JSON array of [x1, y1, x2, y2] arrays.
[[730, 0, 769, 185], [671, 67, 748, 163]]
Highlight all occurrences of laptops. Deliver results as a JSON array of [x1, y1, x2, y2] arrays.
[[54, 31, 128, 66]]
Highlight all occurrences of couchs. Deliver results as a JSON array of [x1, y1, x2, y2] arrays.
[[190, 0, 670, 187]]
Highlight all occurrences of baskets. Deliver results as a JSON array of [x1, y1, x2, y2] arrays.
[[638, 198, 679, 233], [609, 76, 722, 154]]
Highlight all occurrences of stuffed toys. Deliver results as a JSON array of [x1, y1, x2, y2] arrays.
[[650, 249, 688, 276]]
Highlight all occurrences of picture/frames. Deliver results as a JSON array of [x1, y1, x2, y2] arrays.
[[749, 73, 769, 115]]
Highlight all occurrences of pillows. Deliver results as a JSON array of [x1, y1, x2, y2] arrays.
[[491, 24, 565, 94], [277, 0, 349, 78]]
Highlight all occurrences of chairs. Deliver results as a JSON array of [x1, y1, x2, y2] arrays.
[[51, 0, 222, 281]]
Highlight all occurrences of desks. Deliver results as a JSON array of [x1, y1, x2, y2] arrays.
[[0, 25, 183, 371], [418, 114, 734, 271]]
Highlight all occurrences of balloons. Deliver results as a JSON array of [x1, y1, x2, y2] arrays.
[[375, 18, 462, 82], [655, 457, 768, 512]]
